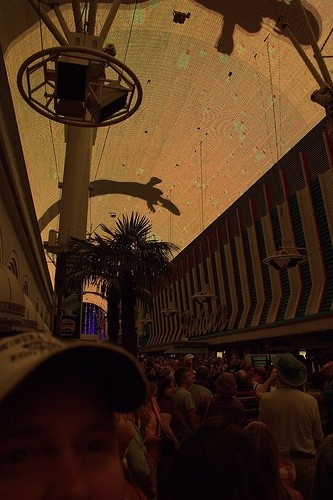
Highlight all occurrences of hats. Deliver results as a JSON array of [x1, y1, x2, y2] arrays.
[[276, 353, 306, 387], [1, 333, 148, 415]]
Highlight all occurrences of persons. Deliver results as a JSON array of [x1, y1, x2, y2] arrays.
[[119, 347, 333, 500], [0, 331, 150, 500]]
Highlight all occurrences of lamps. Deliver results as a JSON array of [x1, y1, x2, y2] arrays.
[[190, 139, 213, 305], [262, 31, 306, 273], [161, 190, 180, 319]]
